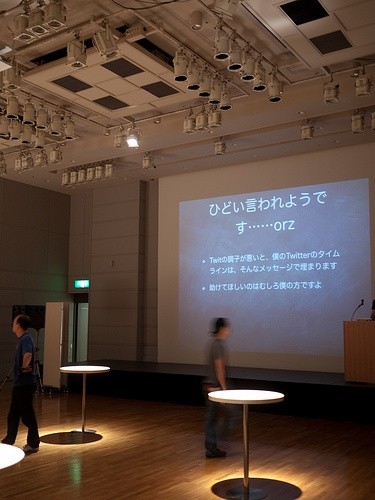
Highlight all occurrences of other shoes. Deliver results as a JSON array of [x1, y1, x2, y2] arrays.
[[205, 447, 227, 457], [21, 444, 39, 452]]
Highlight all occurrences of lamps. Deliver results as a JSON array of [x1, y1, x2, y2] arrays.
[[0, 0, 375, 189]]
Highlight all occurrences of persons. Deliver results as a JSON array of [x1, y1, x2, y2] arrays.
[[371, 299, 375, 320], [0, 315, 41, 453], [204, 317, 232, 457]]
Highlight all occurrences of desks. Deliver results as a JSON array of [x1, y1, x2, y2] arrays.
[[0, 442, 25, 471], [208, 390, 285, 500], [60, 366, 110, 437]]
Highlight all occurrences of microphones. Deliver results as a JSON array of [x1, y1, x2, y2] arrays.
[[351, 298, 364, 321]]
